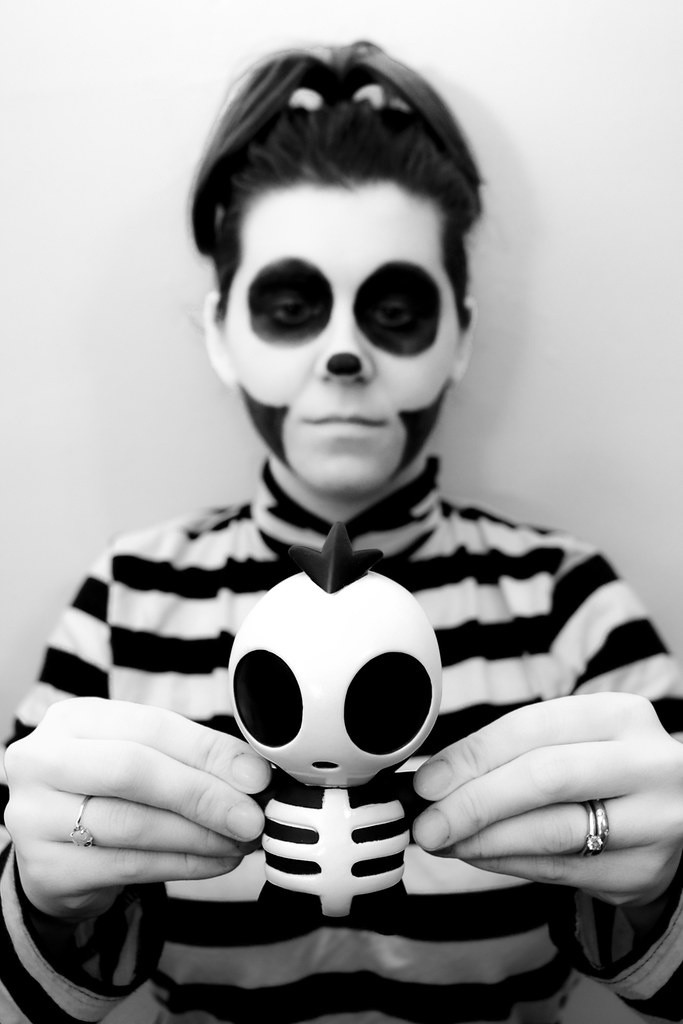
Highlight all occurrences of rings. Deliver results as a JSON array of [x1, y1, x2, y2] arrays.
[[71, 796, 94, 847], [579, 800, 610, 857]]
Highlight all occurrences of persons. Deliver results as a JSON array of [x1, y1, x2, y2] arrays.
[[0, 45, 683, 1024]]
